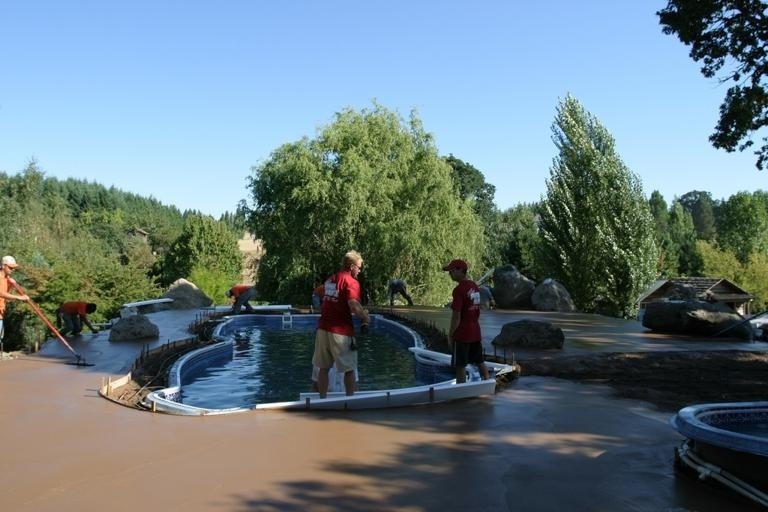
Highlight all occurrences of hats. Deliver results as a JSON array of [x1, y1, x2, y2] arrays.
[[1, 255, 19, 268], [443, 260, 467, 271]]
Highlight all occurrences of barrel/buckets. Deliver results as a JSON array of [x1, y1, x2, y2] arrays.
[[465, 363, 481, 383]]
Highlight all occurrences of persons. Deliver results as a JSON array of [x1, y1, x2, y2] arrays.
[[223, 284, 256, 314], [55, 301, 99, 338], [310, 249, 371, 400], [441, 258, 490, 386], [0, 255, 30, 345], [387, 279, 415, 307]]
